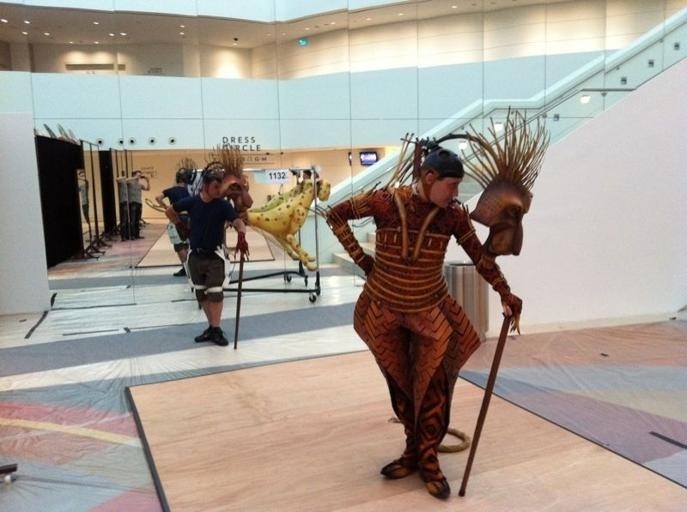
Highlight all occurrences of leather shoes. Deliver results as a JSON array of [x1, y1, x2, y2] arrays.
[[131, 235, 144, 240]]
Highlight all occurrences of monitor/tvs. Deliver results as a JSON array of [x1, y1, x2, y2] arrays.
[[359, 151, 378, 166]]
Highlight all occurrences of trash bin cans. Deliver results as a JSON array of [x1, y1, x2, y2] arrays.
[[444, 261, 489, 343]]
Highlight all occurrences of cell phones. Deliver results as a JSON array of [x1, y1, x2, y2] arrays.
[[140, 175, 145, 179]]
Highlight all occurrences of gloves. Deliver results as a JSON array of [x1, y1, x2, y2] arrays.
[[235, 231, 249, 258], [175, 222, 190, 241], [493, 281, 522, 318]]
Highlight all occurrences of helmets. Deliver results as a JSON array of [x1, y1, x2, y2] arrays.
[[423, 149, 465, 178], [470, 175, 531, 227], [176, 168, 192, 183], [203, 171, 223, 182]]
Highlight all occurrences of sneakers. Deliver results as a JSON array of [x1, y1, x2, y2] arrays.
[[419, 469, 450, 498], [381, 449, 415, 478], [195, 326, 228, 346], [174, 268, 186, 276]]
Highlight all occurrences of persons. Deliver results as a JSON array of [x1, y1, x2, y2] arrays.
[[156, 172, 191, 277], [325, 150, 524, 502], [76, 170, 93, 242], [126, 170, 151, 241], [116, 170, 129, 242], [166, 174, 249, 346]]
